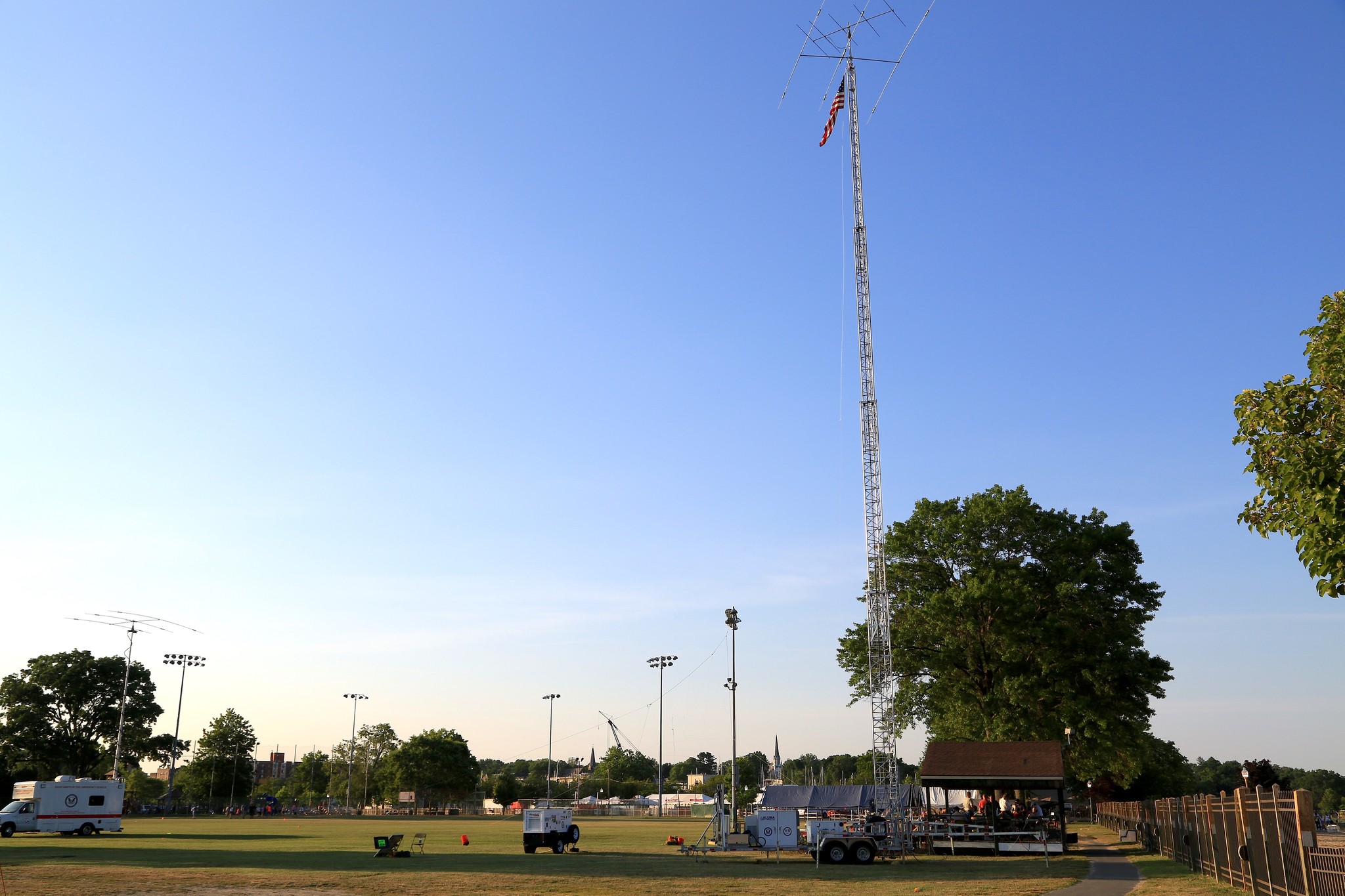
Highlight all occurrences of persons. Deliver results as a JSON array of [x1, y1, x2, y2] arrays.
[[1324, 812, 1331, 825], [1335, 820, 1340, 826], [122, 791, 400, 818], [950, 789, 1042, 827], [1313, 810, 1319, 817], [1319, 815, 1326, 830], [1314, 812, 1321, 830]]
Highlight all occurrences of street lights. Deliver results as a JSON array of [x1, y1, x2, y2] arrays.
[[543, 693, 562, 806], [343, 693, 370, 812], [162, 651, 206, 816], [646, 653, 678, 816]]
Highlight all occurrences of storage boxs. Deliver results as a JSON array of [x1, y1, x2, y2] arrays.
[[1326, 825, 1338, 833]]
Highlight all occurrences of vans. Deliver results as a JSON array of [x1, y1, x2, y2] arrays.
[[141, 804, 158, 811]]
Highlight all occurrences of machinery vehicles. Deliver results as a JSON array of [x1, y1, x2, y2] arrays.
[[676, 2, 1053, 870]]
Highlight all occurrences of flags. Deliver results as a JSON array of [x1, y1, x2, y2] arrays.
[[819, 76, 844, 147]]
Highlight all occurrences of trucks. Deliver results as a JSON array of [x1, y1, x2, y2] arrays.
[[0, 775, 125, 838], [523, 809, 580, 854]]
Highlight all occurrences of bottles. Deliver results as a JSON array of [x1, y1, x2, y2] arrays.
[[419, 837, 422, 844]]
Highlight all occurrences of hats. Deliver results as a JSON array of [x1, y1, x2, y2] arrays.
[[1031, 802, 1036, 807], [955, 806, 959, 810], [922, 811, 925, 815], [1015, 798, 1019, 804]]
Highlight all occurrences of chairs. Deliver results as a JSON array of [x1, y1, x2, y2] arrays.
[[933, 814, 1050, 841], [409, 833, 426, 855], [389, 834, 404, 854]]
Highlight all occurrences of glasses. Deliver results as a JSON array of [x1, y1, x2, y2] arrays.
[[923, 814, 926, 815]]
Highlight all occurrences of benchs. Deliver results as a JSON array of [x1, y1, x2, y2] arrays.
[[282, 806, 351, 816], [386, 812, 409, 815]]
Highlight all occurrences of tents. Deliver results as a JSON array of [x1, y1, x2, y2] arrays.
[[570, 790, 714, 807], [759, 782, 982, 821]]
